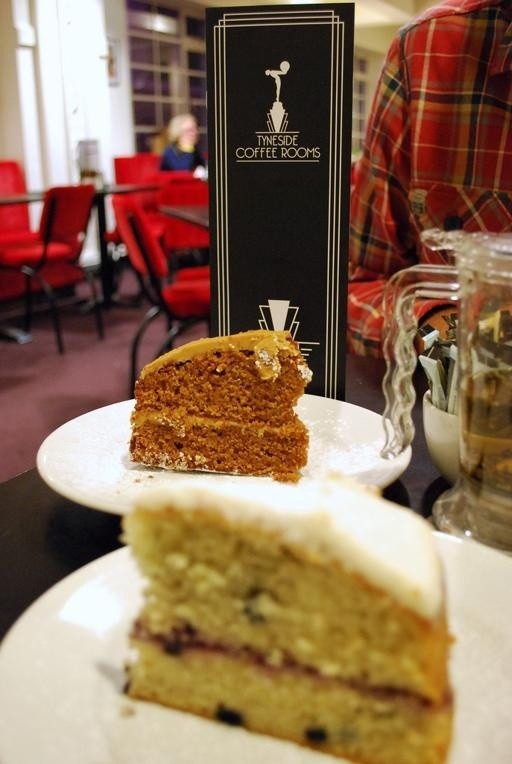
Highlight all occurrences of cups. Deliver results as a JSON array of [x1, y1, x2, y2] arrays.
[[382, 226, 510, 560]]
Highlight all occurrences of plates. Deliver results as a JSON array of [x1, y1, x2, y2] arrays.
[[0, 526, 512, 764], [29, 385, 414, 521]]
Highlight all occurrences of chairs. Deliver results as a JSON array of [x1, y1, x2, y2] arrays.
[[0, 153, 214, 397]]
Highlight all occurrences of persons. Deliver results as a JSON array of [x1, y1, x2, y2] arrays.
[[157, 111, 205, 170], [345, 1, 512, 371]]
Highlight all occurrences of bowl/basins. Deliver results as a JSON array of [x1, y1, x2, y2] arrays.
[[418, 391, 466, 489]]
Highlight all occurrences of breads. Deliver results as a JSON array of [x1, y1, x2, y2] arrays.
[[106, 482, 456, 763], [130, 330, 311, 484]]
